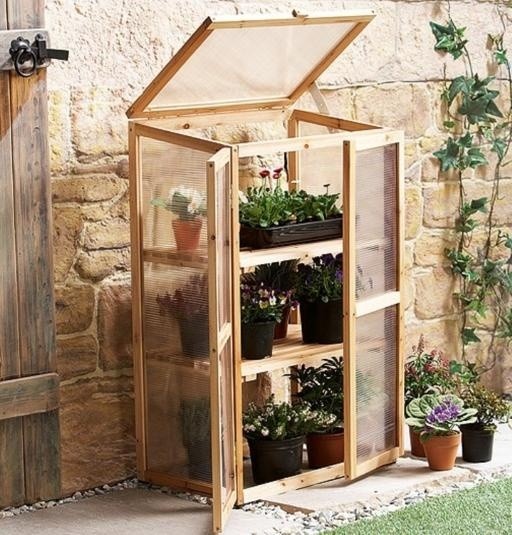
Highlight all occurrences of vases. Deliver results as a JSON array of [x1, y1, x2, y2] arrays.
[[409, 424, 495, 471], [243, 320, 275, 360], [179, 319, 209, 357], [189, 441, 210, 481], [171, 220, 201, 250], [248, 436, 304, 484], [300, 301, 344, 343]]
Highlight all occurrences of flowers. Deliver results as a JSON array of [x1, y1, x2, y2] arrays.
[[148, 184, 210, 220], [405, 334, 511, 443], [241, 394, 334, 440], [156, 270, 209, 320], [239, 281, 295, 323], [296, 253, 372, 303], [180, 397, 212, 445]]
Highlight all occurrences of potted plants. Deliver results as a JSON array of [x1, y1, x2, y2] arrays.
[[241, 259, 302, 339], [239, 169, 343, 249], [281, 356, 377, 470]]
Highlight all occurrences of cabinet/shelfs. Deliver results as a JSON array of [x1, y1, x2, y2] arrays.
[[128, 104, 404, 507]]
